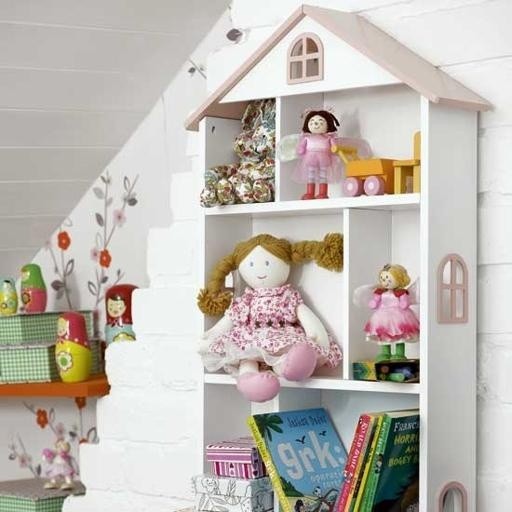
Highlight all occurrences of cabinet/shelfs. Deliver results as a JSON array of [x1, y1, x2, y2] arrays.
[[181, 1, 497, 511]]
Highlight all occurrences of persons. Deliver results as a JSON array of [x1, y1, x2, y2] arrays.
[[353, 263, 420, 360], [195, 234, 344, 403], [297, 105, 340, 201]]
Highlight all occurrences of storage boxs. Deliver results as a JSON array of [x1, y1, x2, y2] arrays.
[[0, 339, 103, 384], [190, 472, 274, 511], [0, 309, 94, 346], [0, 476, 85, 512]]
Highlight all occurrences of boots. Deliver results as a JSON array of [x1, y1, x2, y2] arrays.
[[315, 183, 329, 198], [376, 345, 390, 361], [302, 183, 315, 199], [390, 343, 406, 359]]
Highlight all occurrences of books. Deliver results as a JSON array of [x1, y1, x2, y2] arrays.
[[246, 407, 348, 512], [334, 408, 422, 512]]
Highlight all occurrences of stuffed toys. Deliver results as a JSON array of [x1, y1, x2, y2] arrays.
[[200, 98, 276, 208], [43, 441, 79, 491]]
